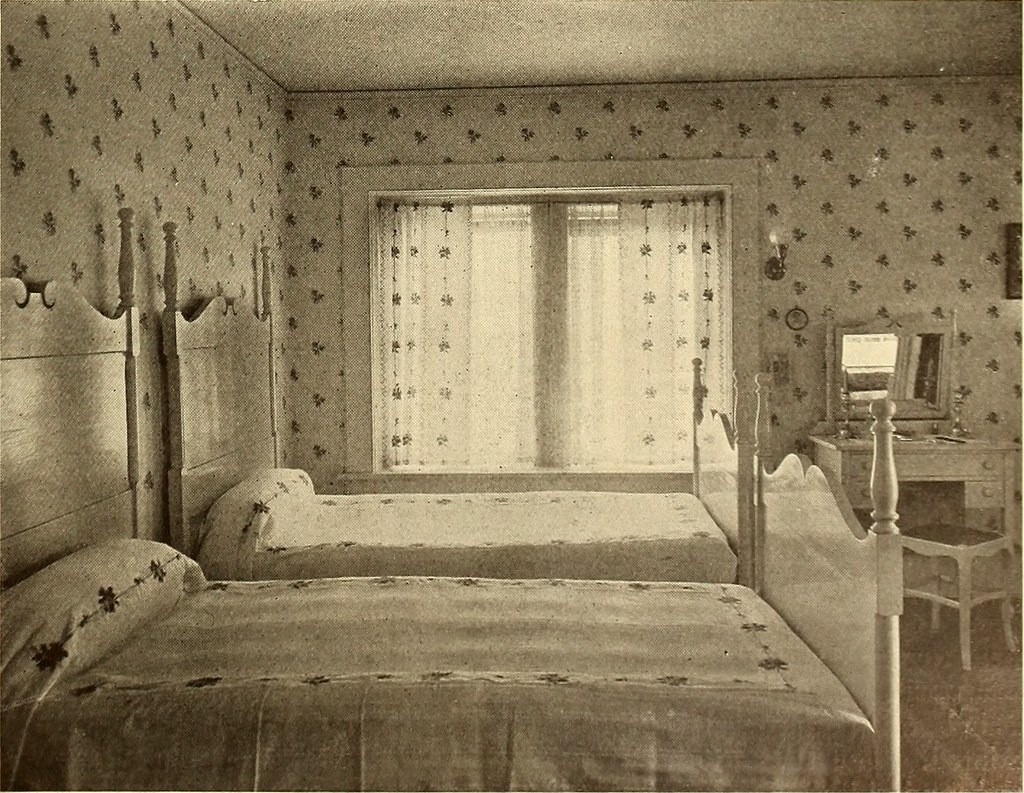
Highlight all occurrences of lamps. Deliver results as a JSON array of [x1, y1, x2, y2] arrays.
[[766, 223, 790, 281]]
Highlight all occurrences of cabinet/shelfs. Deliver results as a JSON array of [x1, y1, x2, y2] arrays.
[[807, 432, 1022, 540]]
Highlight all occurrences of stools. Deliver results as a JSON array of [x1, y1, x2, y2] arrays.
[[903, 525, 1018, 668]]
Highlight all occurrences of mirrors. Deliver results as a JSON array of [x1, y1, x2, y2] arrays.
[[841, 330, 945, 418]]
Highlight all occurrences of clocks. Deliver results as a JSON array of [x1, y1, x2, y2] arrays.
[[786, 303, 808, 331]]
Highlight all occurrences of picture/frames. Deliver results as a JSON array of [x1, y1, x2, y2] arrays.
[[1003, 224, 1022, 301]]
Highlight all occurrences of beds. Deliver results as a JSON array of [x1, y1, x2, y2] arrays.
[[163, 223, 747, 580], [1, 207, 902, 791]]
